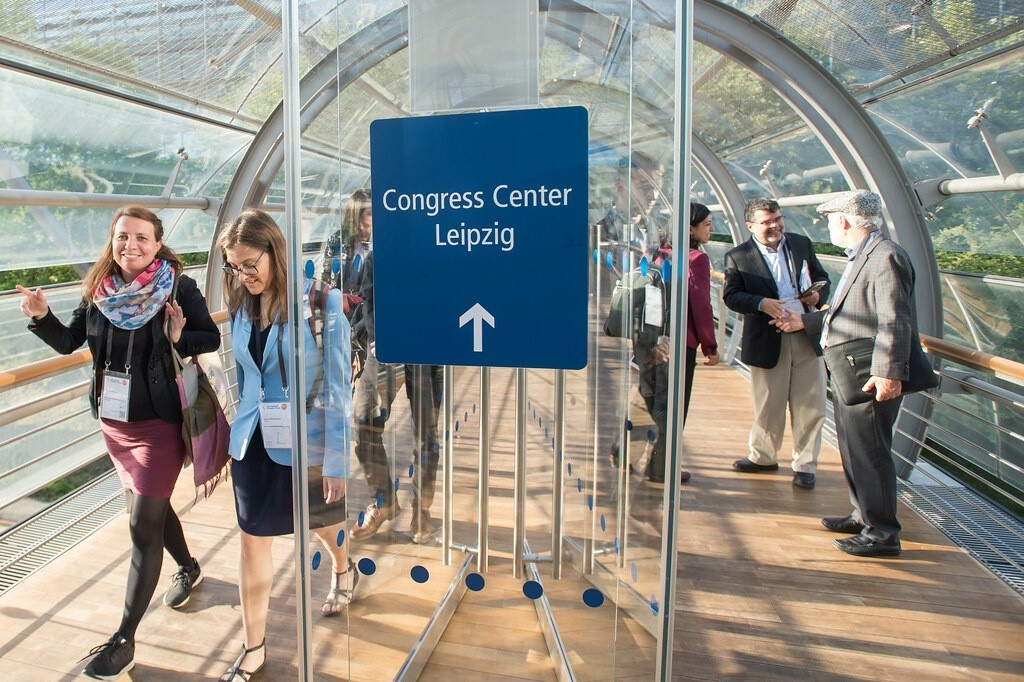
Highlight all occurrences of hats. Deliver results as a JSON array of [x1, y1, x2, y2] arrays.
[[816, 189, 882, 215]]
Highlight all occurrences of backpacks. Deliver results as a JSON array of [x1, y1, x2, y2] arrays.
[[603, 243, 694, 348], [230, 277, 370, 415]]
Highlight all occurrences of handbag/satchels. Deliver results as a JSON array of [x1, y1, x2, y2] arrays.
[[824, 336, 938, 406], [182, 372, 231, 512]]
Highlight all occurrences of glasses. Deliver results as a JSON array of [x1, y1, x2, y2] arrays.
[[749, 216, 785, 225], [221, 249, 265, 276]]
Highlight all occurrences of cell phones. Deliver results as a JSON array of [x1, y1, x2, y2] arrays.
[[797, 281, 827, 300]]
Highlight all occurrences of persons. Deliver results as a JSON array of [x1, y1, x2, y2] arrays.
[[215, 209, 359, 682], [593, 151, 671, 298], [767, 188, 919, 558], [723, 200, 832, 489], [319, 190, 443, 544], [15, 205, 222, 681], [610, 202, 719, 488]]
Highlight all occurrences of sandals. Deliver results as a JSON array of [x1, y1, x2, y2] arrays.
[[218, 637, 266, 682], [321, 557, 359, 616]]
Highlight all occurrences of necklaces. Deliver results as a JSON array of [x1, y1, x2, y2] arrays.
[[261, 315, 271, 329]]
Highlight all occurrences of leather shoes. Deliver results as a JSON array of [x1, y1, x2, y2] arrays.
[[350, 495, 401, 541], [410, 510, 432, 543], [822, 514, 866, 534], [650, 472, 690, 483], [610, 453, 634, 475], [793, 472, 815, 489], [733, 458, 778, 473], [832, 533, 901, 556]]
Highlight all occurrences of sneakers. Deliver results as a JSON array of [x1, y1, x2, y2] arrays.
[[77, 632, 135, 682], [163, 557, 203, 608]]
